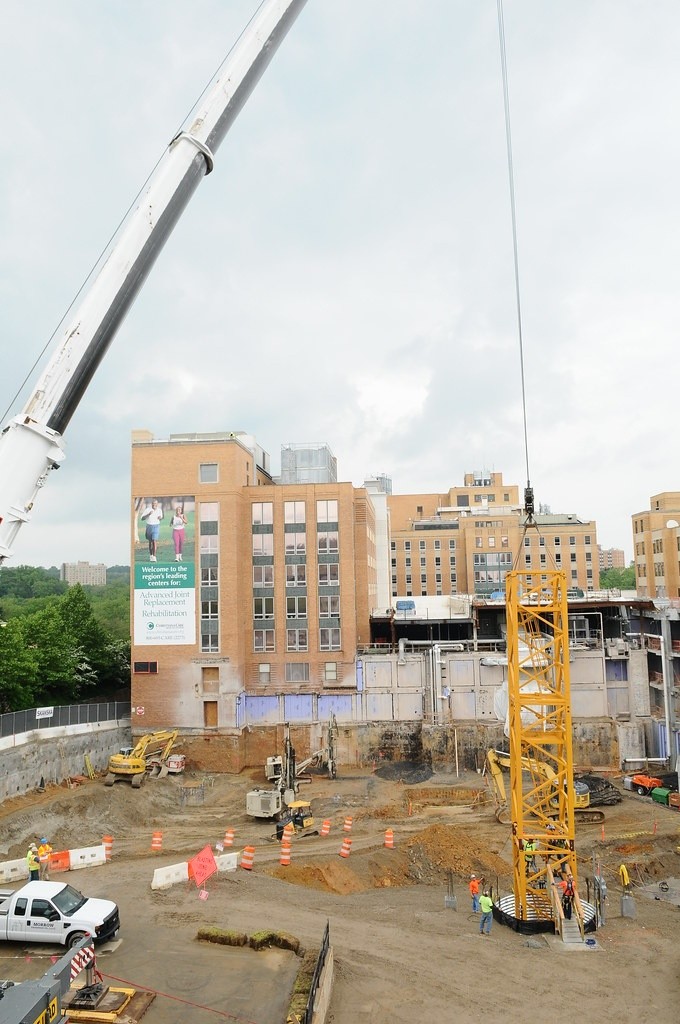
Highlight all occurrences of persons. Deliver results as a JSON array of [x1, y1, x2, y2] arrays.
[[470, 840, 577, 936], [169, 506, 187, 561], [28, 839, 53, 881], [141, 499, 163, 561]]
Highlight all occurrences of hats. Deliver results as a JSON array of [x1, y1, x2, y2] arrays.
[[470, 875, 475, 879], [483, 890, 489, 895]]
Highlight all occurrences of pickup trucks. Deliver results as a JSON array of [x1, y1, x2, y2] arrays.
[[0, 880, 120, 951]]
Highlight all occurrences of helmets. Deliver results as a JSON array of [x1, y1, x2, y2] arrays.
[[28, 842, 36, 848], [31, 847, 38, 851], [40, 838, 47, 844]]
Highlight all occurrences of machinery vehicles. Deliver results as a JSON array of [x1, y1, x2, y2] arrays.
[[0, 0, 542, 560], [486, 748, 591, 830], [245, 722, 312, 822], [266, 709, 340, 782], [104, 728, 182, 790]]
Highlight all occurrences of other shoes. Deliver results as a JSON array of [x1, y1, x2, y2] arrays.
[[480, 932, 485, 935], [485, 933, 491, 936], [473, 910, 476, 912], [476, 911, 480, 914]]
